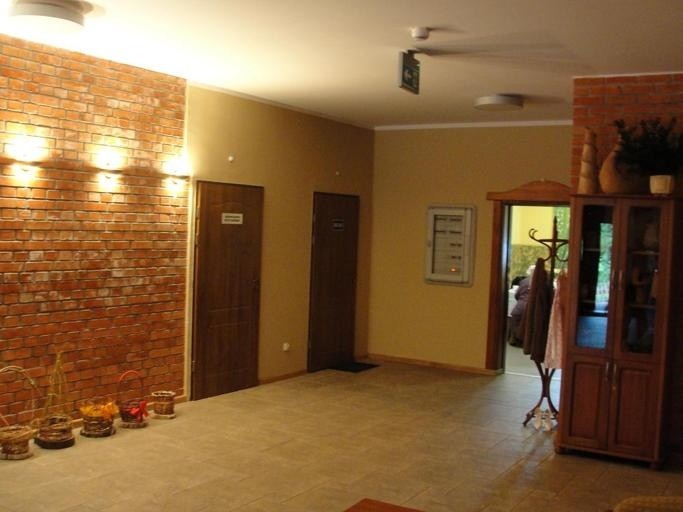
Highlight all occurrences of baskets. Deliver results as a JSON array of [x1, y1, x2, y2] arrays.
[[116, 370, 147, 423]]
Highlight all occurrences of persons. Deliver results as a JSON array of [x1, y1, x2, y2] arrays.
[[507, 264, 536, 348]]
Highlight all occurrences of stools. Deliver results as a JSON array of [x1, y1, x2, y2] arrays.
[[507, 317, 513, 340]]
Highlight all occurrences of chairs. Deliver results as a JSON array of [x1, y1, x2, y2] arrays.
[[516, 288, 529, 336]]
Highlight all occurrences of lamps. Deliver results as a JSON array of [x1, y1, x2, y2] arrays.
[[470, 94, 524, 113], [9, 0, 85, 32]]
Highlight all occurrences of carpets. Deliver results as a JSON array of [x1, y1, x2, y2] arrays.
[[344, 496, 422, 512], [329, 361, 380, 373]]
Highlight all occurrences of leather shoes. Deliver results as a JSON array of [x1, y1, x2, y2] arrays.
[[534, 407, 552, 431]]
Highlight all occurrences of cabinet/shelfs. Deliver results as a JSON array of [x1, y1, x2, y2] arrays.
[[554, 191, 678, 471]]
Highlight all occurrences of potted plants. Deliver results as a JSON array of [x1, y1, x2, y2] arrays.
[[611, 116, 683, 197]]
